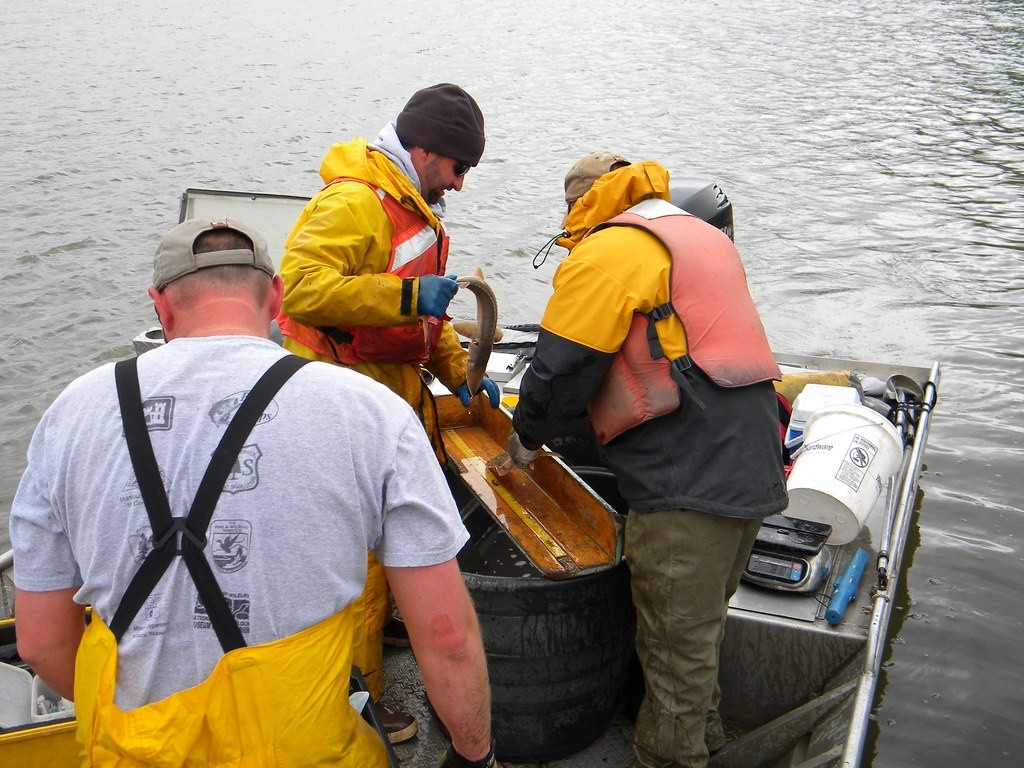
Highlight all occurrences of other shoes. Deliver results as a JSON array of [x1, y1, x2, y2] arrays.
[[382, 616, 413, 650], [368, 698, 418, 744]]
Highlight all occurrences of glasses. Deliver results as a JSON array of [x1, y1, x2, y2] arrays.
[[454, 163, 471, 177]]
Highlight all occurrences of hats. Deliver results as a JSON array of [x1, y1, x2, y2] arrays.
[[396, 82, 487, 166], [152, 216, 274, 290], [562, 151, 634, 204]]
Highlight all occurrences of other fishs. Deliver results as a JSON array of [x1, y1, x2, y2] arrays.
[[418, 265, 497, 400], [486, 447, 563, 477]]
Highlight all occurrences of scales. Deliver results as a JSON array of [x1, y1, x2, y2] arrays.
[[740, 515, 833, 595]]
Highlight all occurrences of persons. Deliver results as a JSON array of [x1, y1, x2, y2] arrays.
[[276, 83, 499, 746], [7, 218, 498, 768], [508, 153, 789, 768]]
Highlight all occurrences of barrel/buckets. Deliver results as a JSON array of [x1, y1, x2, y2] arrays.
[[781, 403, 903, 545]]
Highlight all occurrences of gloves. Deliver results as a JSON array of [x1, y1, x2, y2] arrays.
[[507, 428, 543, 469], [456, 373, 501, 410], [413, 274, 459, 319]]
